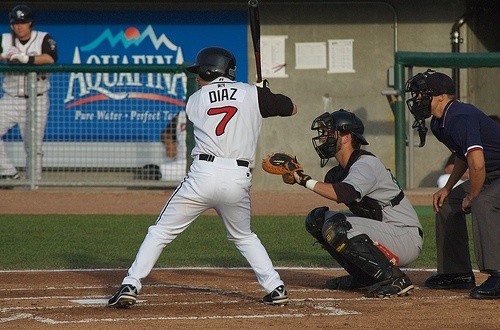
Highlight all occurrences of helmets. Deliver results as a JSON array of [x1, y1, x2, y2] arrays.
[[186, 47, 237, 80], [329, 109, 368, 146], [9, 5, 33, 30]]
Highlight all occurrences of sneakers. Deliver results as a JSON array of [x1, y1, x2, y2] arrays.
[[372, 272, 414, 298], [262, 285, 289, 305], [326, 275, 370, 289], [108, 284, 137, 309]]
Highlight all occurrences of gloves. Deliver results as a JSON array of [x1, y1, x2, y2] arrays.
[[253, 79, 270, 90], [10, 53, 30, 65], [2, 47, 21, 59]]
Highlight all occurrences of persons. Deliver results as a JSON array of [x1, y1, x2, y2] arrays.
[[403, 69, 500, 299], [437, 115, 500, 191], [0, 6, 58, 186], [108, 47, 297, 306], [262, 108, 423, 296], [145, 111, 187, 181]]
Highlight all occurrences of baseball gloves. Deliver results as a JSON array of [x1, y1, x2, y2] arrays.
[[261, 152, 305, 185]]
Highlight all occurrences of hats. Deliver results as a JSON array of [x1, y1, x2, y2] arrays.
[[423, 72, 456, 96]]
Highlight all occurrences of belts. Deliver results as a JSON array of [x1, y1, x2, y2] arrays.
[[419, 228, 423, 239], [18, 94, 42, 98], [199, 154, 249, 167]]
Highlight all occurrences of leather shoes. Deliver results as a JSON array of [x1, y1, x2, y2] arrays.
[[472, 273, 500, 298], [425, 272, 475, 289]]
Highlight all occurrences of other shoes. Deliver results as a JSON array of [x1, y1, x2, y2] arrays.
[[0, 173, 20, 189]]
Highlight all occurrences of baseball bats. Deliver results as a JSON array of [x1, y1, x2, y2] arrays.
[[247, 0, 262, 83]]
